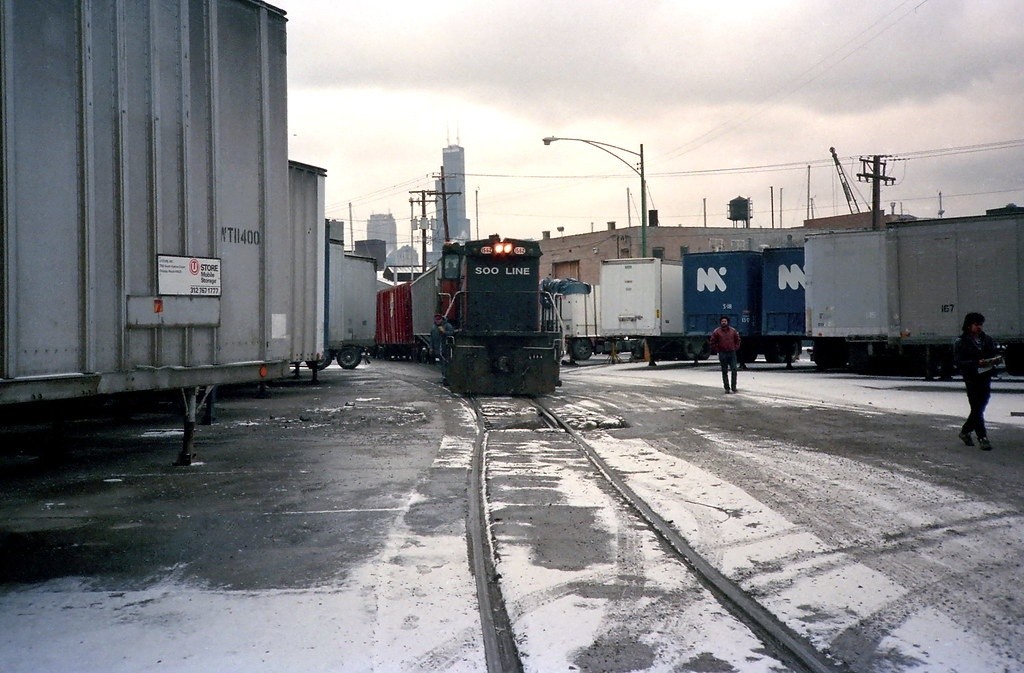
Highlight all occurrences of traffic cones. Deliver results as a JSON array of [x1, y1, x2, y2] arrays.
[[642, 342, 650, 362]]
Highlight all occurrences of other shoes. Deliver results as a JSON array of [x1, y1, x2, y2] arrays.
[[977, 436, 993, 452], [725, 389, 729, 394], [959, 430, 975, 446], [732, 388, 738, 392]]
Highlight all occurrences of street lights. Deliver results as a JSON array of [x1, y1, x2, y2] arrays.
[[542, 136, 647, 259]]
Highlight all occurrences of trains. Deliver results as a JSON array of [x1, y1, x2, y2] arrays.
[[378, 234, 563, 397]]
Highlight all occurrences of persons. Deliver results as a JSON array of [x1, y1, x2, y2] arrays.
[[953, 311, 1000, 451], [431, 314, 455, 381], [709, 316, 741, 394]]
